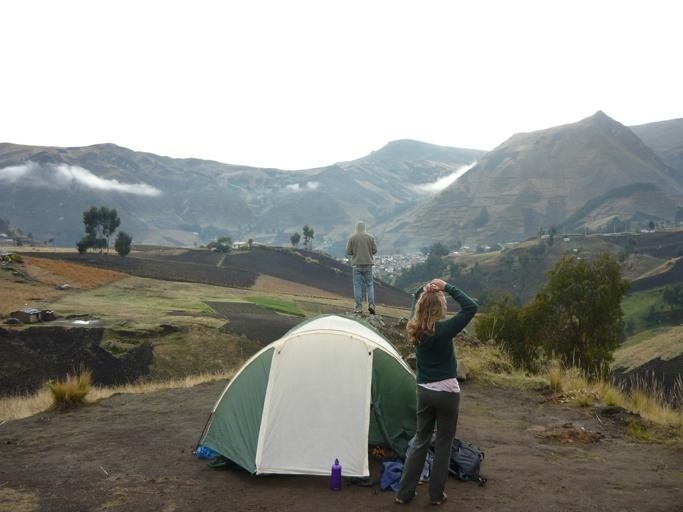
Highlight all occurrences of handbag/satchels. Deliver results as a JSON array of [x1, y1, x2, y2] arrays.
[[448, 438, 487, 486]]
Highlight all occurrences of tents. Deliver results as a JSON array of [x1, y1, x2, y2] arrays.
[[199, 314, 416, 478]]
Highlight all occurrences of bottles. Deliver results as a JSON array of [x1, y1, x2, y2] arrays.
[[329, 458, 341, 491], [196, 445, 214, 457]]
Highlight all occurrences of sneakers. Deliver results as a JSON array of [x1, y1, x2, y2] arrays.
[[353, 309, 362, 313], [432, 491, 448, 505], [368, 303, 376, 314], [394, 492, 418, 503]]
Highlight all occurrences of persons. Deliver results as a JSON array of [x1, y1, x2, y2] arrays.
[[345, 222, 377, 315], [395, 278, 478, 505]]
